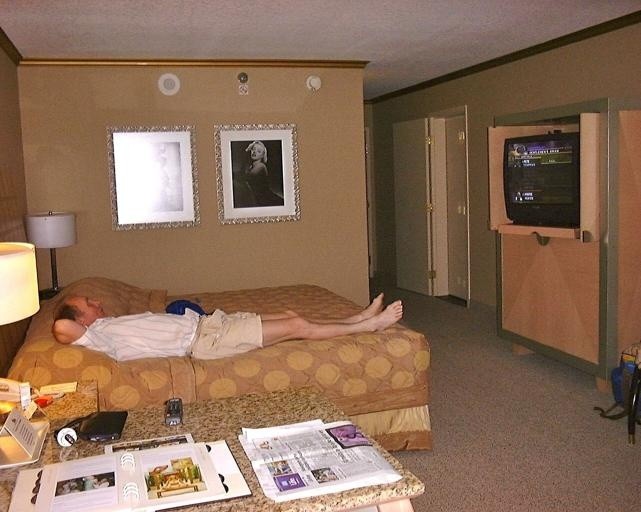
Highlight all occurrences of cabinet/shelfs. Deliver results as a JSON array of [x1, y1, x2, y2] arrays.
[[488, 97, 621, 392]]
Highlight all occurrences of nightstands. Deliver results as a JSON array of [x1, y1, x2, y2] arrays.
[[29, 379, 99, 420]]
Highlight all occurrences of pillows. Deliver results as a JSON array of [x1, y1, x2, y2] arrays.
[[5, 336, 112, 389], [48, 278, 128, 318]]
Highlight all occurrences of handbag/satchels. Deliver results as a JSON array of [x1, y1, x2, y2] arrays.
[[165, 299, 207, 316]]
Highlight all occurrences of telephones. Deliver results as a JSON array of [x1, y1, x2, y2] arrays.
[[0, 378, 37, 402]]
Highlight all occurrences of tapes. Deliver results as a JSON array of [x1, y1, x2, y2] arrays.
[[56, 428, 76, 447]]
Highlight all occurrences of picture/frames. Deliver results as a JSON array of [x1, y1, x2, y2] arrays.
[[213, 124, 301, 225], [104, 124, 201, 232]]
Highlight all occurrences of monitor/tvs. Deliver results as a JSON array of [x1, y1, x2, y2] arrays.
[[503, 132, 580, 228]]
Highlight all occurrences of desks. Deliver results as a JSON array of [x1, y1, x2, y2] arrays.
[[0, 388, 425, 512]]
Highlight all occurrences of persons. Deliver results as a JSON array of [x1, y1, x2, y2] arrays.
[[244, 141, 283, 206], [51, 291, 403, 360]]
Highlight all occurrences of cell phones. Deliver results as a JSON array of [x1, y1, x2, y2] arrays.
[[164, 396, 183, 425]]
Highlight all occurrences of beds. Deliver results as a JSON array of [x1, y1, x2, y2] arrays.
[[0, 285, 433, 453]]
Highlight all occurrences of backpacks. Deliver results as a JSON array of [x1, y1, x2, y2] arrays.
[[593, 340, 641, 423]]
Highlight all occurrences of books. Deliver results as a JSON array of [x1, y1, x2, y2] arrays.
[[7, 439, 253, 512]]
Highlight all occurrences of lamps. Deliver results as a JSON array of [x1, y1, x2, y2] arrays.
[[0, 241, 40, 414], [26, 211, 76, 299]]
